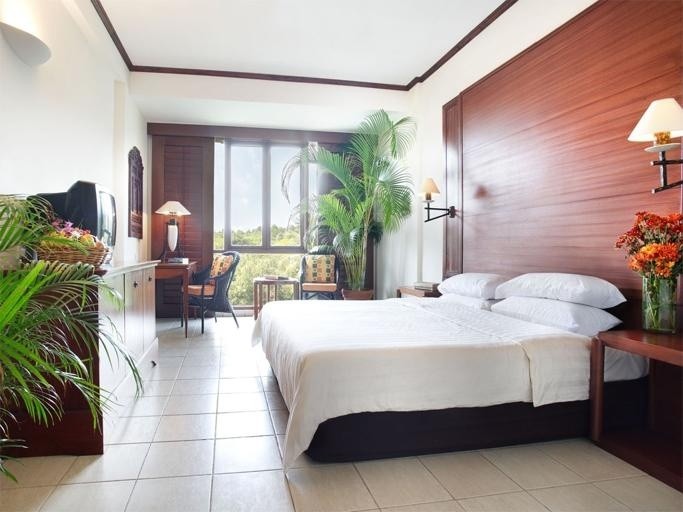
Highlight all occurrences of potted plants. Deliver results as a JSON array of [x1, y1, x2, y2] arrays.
[[281, 109, 418, 299]]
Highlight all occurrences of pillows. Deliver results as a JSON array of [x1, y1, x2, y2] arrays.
[[209, 255, 233, 285], [305, 255, 336, 283], [438, 272, 627, 337]]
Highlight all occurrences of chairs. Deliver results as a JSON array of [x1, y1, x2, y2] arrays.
[[300, 247, 341, 300], [178, 251, 241, 334]]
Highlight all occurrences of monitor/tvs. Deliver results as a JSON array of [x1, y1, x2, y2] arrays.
[[25, 181, 116, 263]]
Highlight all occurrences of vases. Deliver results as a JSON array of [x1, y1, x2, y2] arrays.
[[641, 277, 675, 335]]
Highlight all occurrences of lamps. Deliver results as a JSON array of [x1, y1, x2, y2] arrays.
[[154, 200, 192, 259], [420, 177, 454, 222], [629, 97, 683, 194]]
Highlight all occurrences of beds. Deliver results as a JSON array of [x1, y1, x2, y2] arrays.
[[252, 297, 648, 462]]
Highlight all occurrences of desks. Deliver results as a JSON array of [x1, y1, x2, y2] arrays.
[[155, 261, 197, 339], [253, 278, 299, 320]]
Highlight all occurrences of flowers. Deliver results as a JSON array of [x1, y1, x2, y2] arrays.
[[615, 212, 682, 327]]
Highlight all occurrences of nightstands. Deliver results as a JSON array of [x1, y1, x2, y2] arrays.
[[397, 288, 441, 297], [588, 328, 683, 493]]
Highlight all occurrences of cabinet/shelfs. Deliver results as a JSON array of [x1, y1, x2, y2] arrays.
[[0, 260, 161, 457]]
